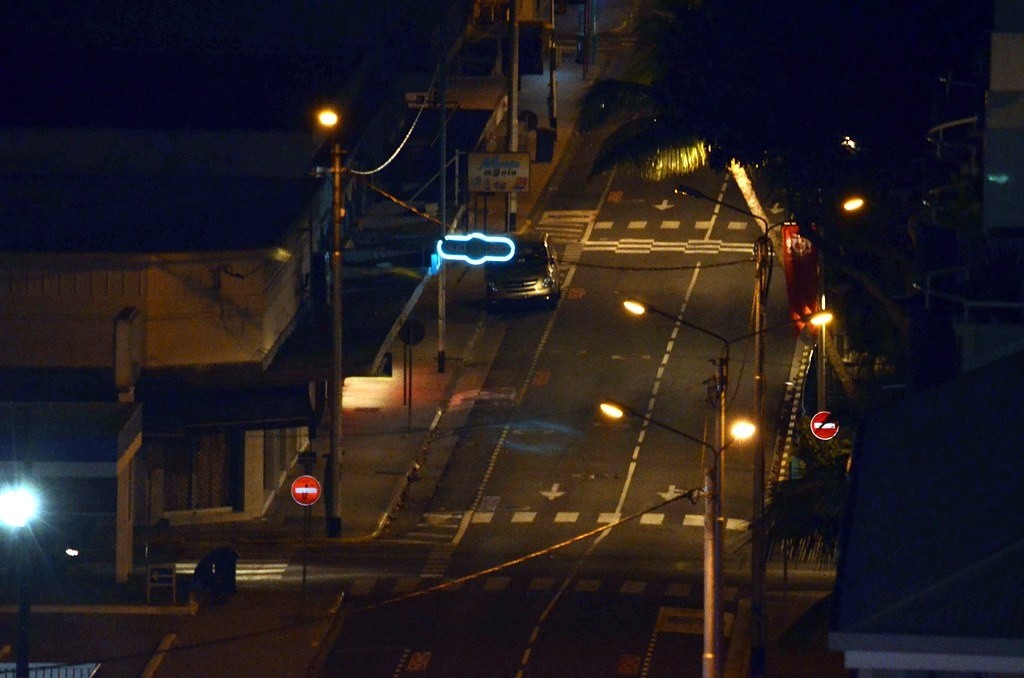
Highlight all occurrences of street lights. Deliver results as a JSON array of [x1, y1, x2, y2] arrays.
[[677, 184, 866, 415], [315, 106, 345, 538], [598, 295, 835, 678], [108, 305, 141, 589], [5, 472, 33, 678]]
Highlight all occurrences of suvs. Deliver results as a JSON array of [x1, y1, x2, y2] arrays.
[[486, 230, 561, 311]]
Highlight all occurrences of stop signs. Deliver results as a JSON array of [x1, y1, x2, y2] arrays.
[[810, 411, 840, 440], [290, 474, 322, 506]]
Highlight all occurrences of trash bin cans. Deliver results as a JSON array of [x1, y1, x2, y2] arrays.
[[534, 127, 556, 162], [214, 548, 239, 593]]
